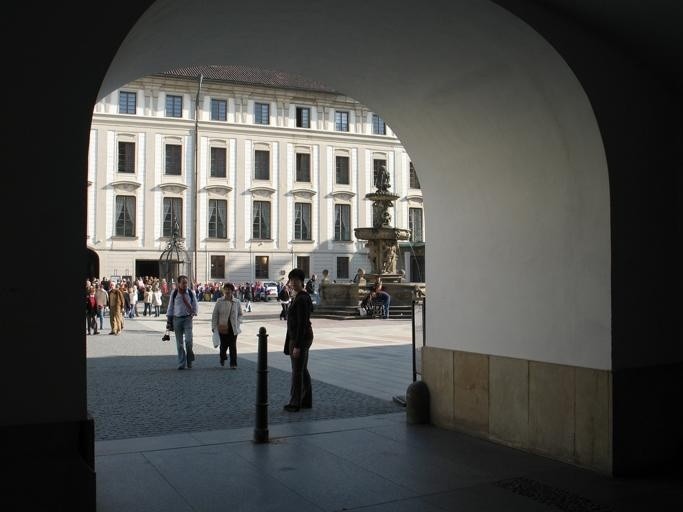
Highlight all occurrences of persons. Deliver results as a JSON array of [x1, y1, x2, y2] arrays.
[[192, 269, 330, 322], [85, 273, 175, 336], [210, 283, 242, 369], [165, 275, 199, 372], [280, 267, 313, 412], [352, 267, 390, 318]]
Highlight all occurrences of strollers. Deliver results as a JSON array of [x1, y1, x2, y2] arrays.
[[368, 297, 386, 319]]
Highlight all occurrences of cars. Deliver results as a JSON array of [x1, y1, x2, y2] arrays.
[[248, 280, 280, 300]]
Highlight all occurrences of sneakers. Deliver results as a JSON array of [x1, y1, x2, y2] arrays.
[[219, 359, 224, 366], [284, 404, 312, 412], [230, 365, 236, 368], [187, 359, 192, 369], [88, 332, 115, 335]]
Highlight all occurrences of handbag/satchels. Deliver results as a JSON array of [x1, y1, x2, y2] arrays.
[[211, 325, 220, 348]]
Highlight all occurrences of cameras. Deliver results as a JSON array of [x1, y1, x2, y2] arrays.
[[162, 335, 170, 341]]
